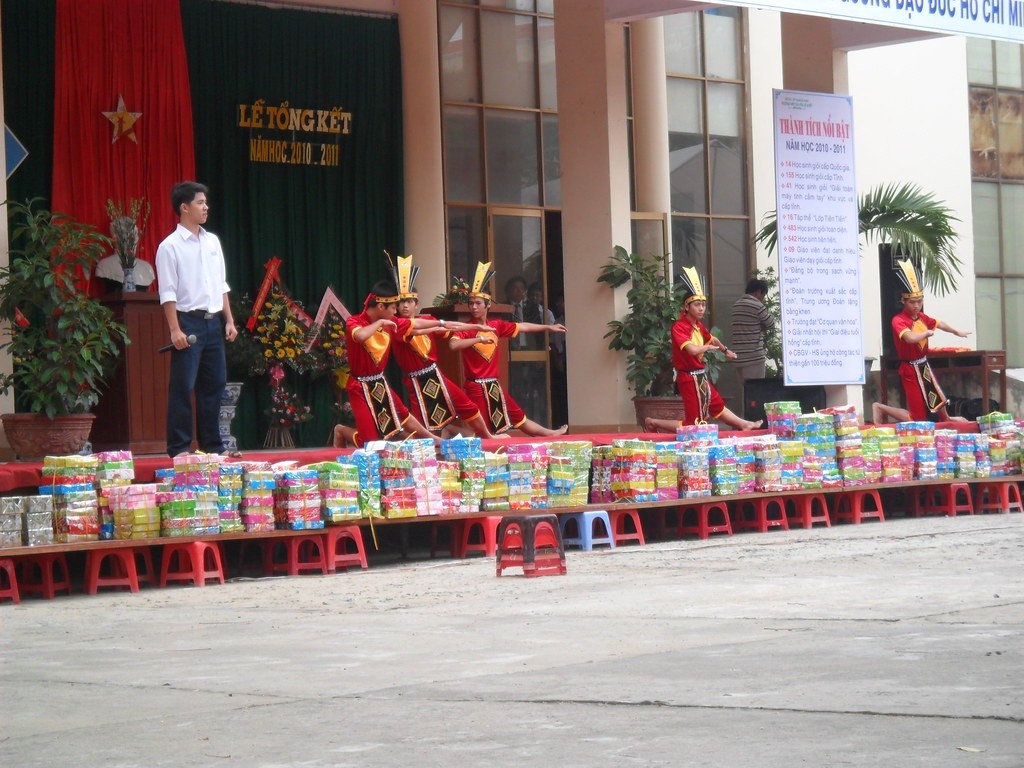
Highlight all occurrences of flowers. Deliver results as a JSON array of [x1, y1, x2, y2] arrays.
[[432, 274, 471, 307], [270, 385, 314, 428], [329, 399, 356, 425], [308, 306, 349, 372], [249, 283, 309, 376]]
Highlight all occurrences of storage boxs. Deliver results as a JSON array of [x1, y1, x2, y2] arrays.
[[0, 399, 1024, 546]]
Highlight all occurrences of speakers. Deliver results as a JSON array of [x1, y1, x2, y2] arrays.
[[744, 378, 827, 430], [879, 242, 922, 362]]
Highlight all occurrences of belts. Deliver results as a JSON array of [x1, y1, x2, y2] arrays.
[[193, 310, 221, 319]]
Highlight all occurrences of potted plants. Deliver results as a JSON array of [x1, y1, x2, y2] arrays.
[[0, 196, 132, 465], [593, 242, 726, 433]]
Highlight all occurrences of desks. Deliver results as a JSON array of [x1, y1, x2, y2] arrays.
[[879, 349, 1007, 423], [615, 487, 844, 510], [843, 474, 1024, 492], [0, 527, 330, 555], [323, 502, 615, 525]]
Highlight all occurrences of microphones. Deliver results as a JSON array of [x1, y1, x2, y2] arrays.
[[159, 335, 197, 353]]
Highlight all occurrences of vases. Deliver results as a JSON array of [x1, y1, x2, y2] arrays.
[[121, 268, 137, 293]]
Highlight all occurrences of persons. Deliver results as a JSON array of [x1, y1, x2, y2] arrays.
[[155, 181, 243, 459], [731, 282, 776, 379], [390, 254, 511, 439], [871, 259, 972, 425], [96, 217, 154, 286], [645, 267, 763, 432], [502, 276, 567, 426], [333, 284, 463, 448], [448, 262, 568, 436]]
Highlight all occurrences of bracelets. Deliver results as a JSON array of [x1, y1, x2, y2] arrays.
[[439, 320, 444, 327], [480, 336, 483, 343], [723, 348, 727, 353]]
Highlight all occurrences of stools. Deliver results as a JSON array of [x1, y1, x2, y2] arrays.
[[6, 552, 75, 599], [263, 524, 369, 580], [925, 484, 952, 517], [734, 496, 790, 533], [110, 546, 157, 591], [599, 509, 646, 546], [832, 489, 885, 525], [461, 516, 502, 558], [947, 483, 974, 517], [906, 485, 936, 518], [676, 502, 733, 539], [976, 481, 1023, 515], [83, 547, 140, 595], [650, 508, 678, 541], [0, 555, 21, 605], [430, 520, 464, 559], [778, 493, 831, 529], [160, 541, 224, 587], [496, 514, 567, 577], [559, 510, 615, 552]]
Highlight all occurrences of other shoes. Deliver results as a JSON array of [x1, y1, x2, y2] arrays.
[[219, 449, 243, 458]]
